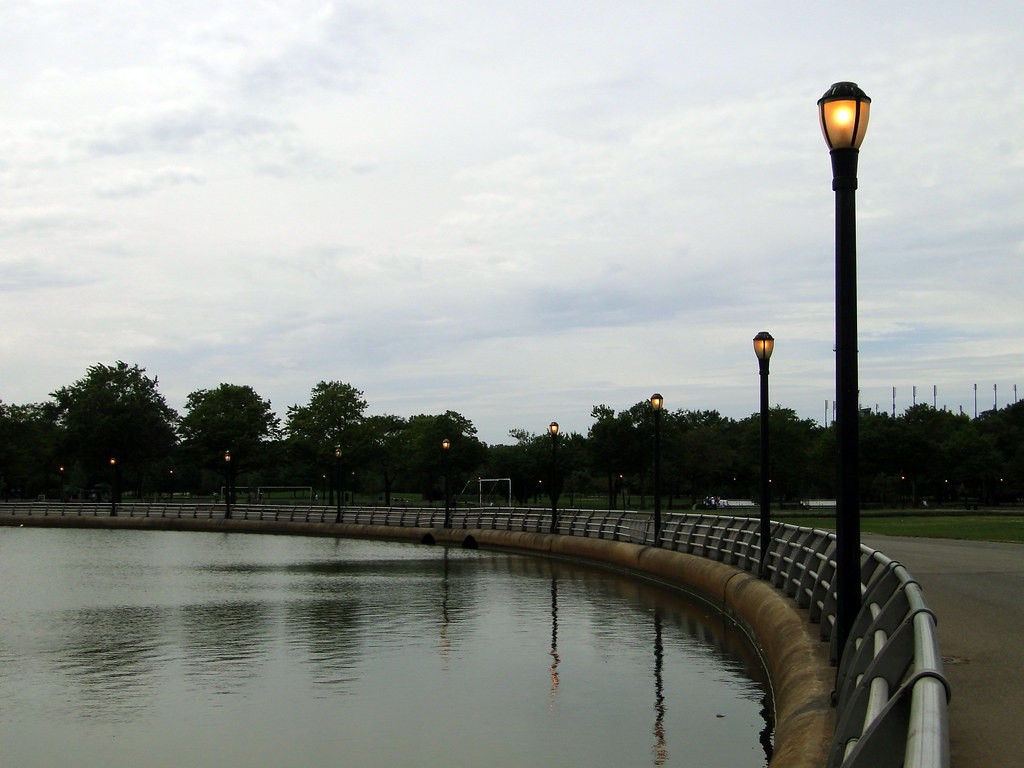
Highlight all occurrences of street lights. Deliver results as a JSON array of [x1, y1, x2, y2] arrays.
[[815, 81, 872, 686], [334, 447, 342, 522], [440, 437, 451, 526], [223, 450, 232, 519], [109, 457, 117, 514], [649, 392, 665, 539], [549, 420, 560, 532], [58, 465, 64, 501], [752, 331, 776, 575]]
